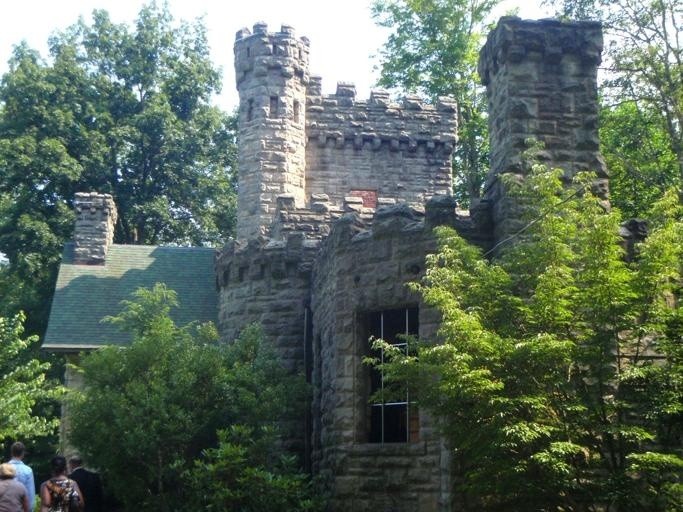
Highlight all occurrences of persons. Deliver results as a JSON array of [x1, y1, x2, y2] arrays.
[[6, 441, 35, 512], [0, 462, 29, 511], [38, 454, 84, 511], [65, 455, 104, 511]]
